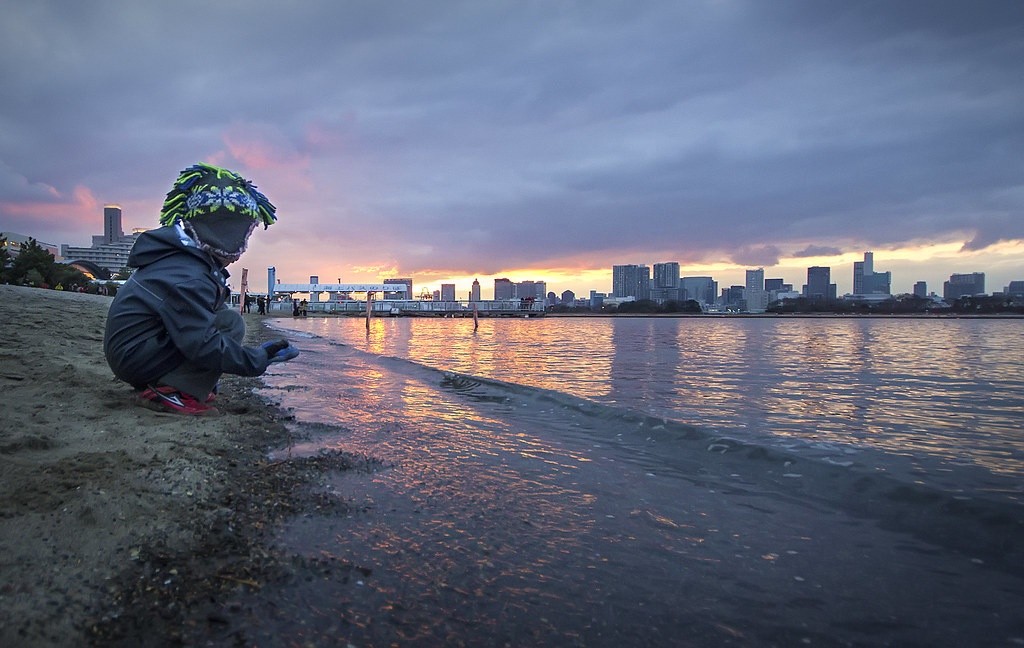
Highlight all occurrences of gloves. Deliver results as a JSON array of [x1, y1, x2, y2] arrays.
[[262, 339, 300, 364]]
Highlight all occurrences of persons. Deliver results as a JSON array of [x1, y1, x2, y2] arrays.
[[96, 284, 110, 296], [103, 162, 300, 417], [54, 281, 63, 290], [520, 296, 534, 311], [244, 292, 273, 315], [293, 298, 308, 316]]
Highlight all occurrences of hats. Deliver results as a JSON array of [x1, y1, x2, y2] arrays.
[[160, 166, 277, 257]]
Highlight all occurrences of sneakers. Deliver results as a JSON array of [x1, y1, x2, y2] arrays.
[[203, 391, 216, 402], [137, 386, 220, 418]]
